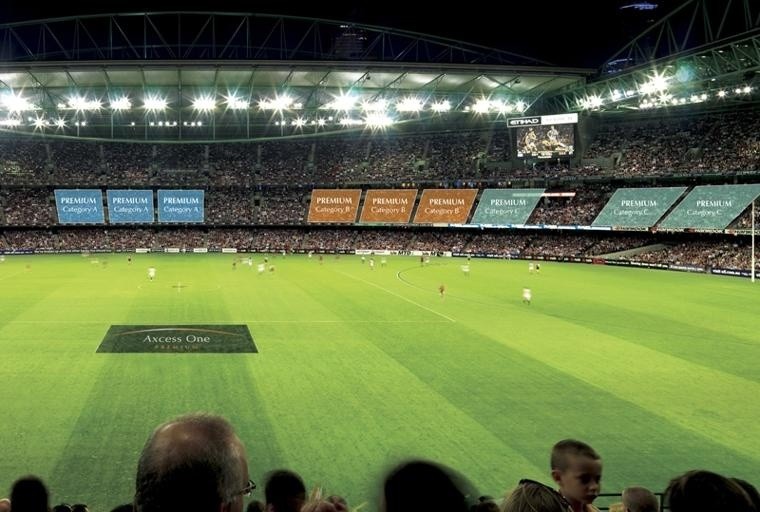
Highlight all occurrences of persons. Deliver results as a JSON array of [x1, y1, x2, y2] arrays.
[[232, 255, 275, 273], [149, 266, 156, 281], [308, 255, 386, 270], [510, 160, 759, 270], [1, 476, 133, 512], [522, 260, 541, 304], [382, 459, 570, 512], [1, 130, 511, 254], [551, 439, 602, 512], [128, 256, 131, 263], [608, 469, 760, 511], [517, 126, 574, 157], [133, 413, 257, 512], [246, 470, 348, 512], [419, 255, 472, 295], [585, 109, 759, 160]]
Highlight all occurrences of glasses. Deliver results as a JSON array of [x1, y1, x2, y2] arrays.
[[229, 480, 256, 500]]
